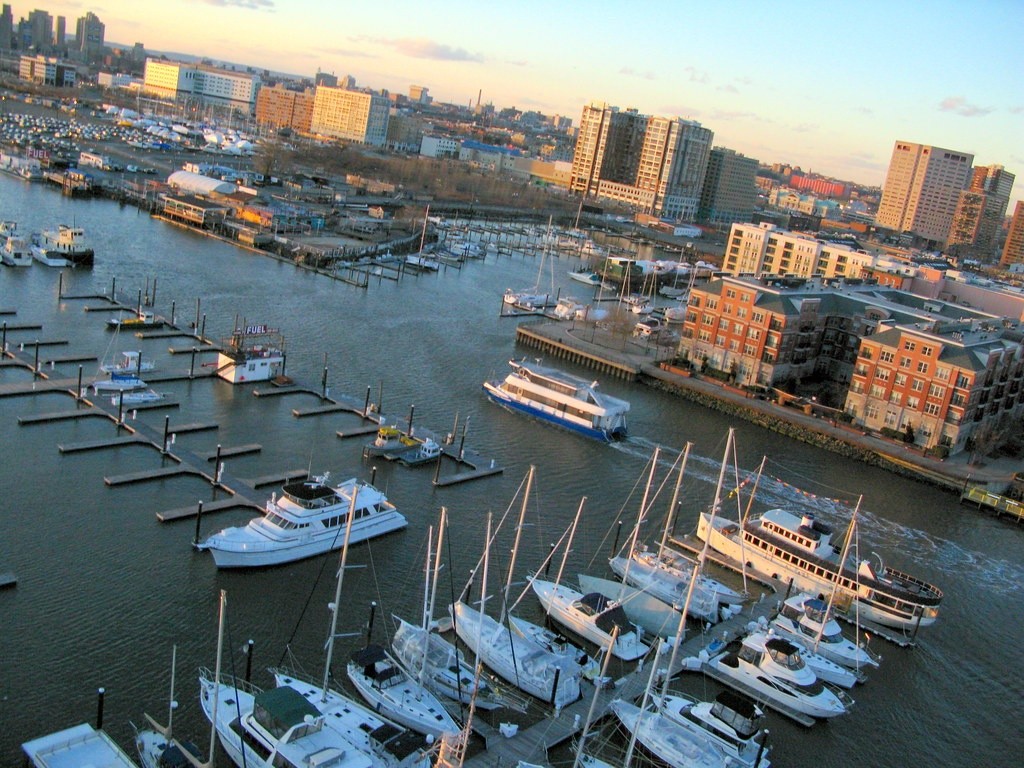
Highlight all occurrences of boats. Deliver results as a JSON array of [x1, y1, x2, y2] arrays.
[[364, 425, 418, 458], [31, 244, 67, 266], [102, 349, 155, 374], [0, 112, 174, 181], [0, 219, 32, 268], [695, 506, 944, 632], [399, 436, 442, 467], [481, 357, 631, 444], [197, 470, 410, 570], [29, 223, 94, 269], [335, 215, 719, 336], [110, 389, 166, 406]]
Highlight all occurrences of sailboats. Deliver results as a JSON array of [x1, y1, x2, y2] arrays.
[[20, 424, 880, 768], [99, 86, 300, 157], [90, 309, 147, 393]]
